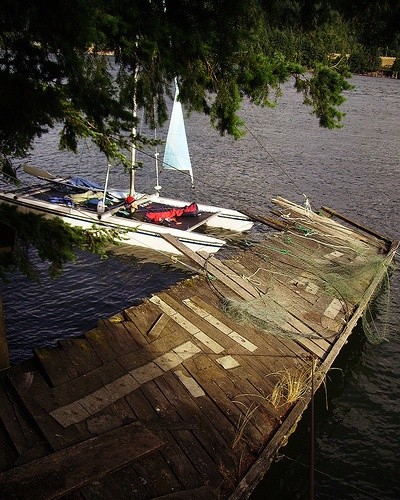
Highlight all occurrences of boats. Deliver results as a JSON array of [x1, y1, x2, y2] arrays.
[[0, 76, 254, 255]]
